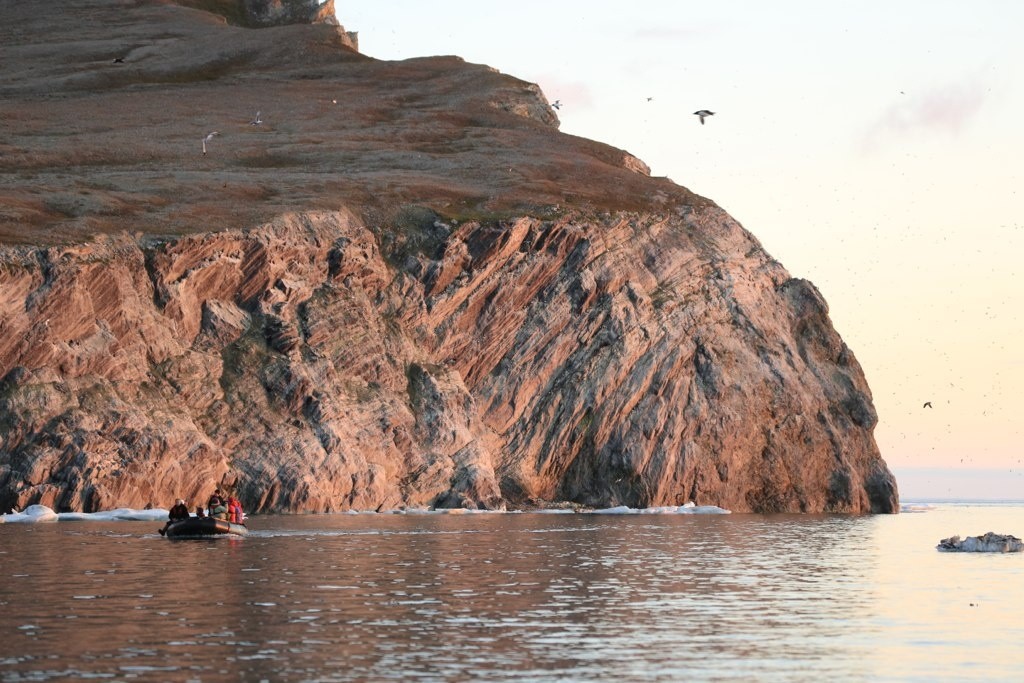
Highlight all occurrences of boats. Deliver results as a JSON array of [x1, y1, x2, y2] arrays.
[[166, 511, 251, 538]]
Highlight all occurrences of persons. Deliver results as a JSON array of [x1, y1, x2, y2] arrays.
[[158, 499, 190, 536], [208, 490, 243, 524], [196, 507, 205, 518], [147, 498, 159, 510]]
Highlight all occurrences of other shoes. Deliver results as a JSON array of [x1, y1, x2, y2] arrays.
[[159, 529, 165, 536]]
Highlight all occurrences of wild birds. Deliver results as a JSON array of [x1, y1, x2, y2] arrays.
[[647, 97, 653, 102], [250, 111, 263, 127], [923, 402, 932, 409], [202, 131, 221, 156], [331, 97, 338, 104], [551, 100, 563, 110], [693, 110, 717, 124]]
